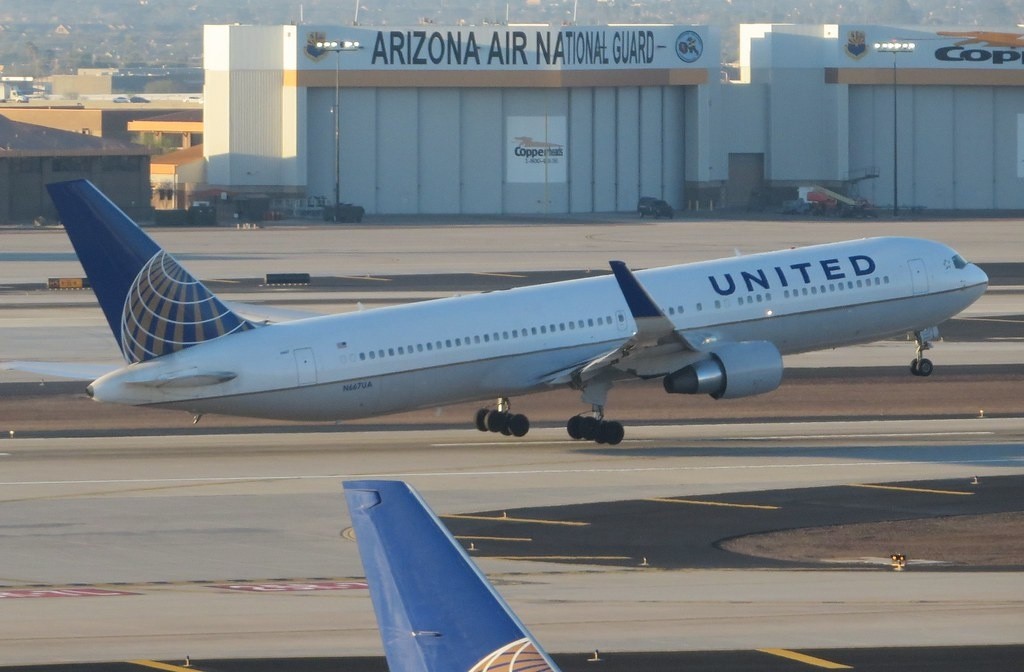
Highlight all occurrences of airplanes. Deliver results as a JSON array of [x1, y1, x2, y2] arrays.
[[0, 174, 994, 446]]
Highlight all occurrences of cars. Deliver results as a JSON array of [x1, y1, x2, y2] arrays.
[[182, 96, 203, 105], [113, 96, 130, 103], [130, 97, 151, 104]]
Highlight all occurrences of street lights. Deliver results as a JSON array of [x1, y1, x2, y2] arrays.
[[315, 41, 360, 207]]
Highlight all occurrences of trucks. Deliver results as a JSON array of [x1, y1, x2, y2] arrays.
[[0, 82, 29, 104]]
